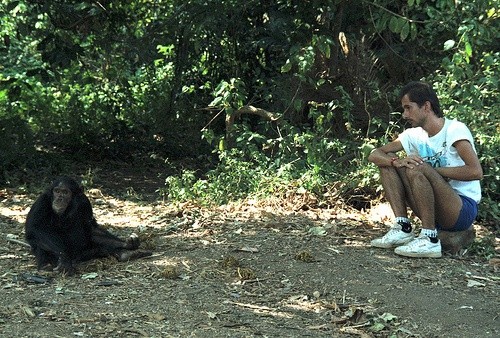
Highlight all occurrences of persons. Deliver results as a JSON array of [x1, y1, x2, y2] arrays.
[[368, 82, 483, 258]]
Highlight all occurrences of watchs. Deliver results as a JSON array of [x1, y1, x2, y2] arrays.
[[391, 157, 400, 167]]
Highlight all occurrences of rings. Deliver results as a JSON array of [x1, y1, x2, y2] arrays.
[[399, 165, 402, 167]]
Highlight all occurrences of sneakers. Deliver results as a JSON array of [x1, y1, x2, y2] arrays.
[[371, 222, 415, 248], [394, 233, 442, 258]]
